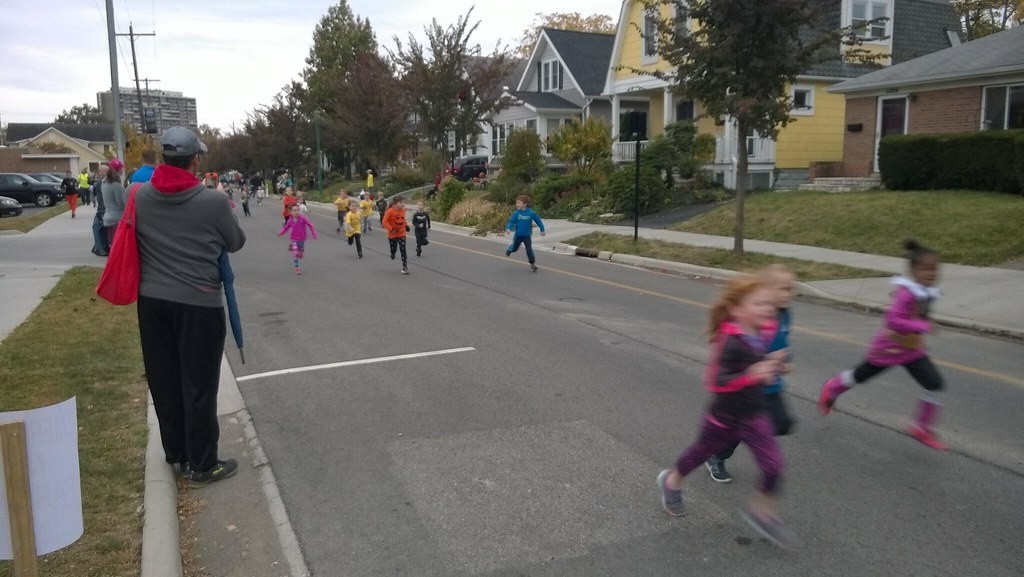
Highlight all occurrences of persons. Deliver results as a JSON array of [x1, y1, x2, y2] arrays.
[[241, 187, 251, 217], [126, 125, 247, 487], [92, 159, 126, 256], [434, 159, 450, 189], [88, 171, 98, 207], [278, 204, 318, 274], [382, 194, 431, 274], [367, 169, 374, 194], [656, 261, 796, 548], [224, 183, 233, 200], [257, 186, 265, 206], [76, 168, 90, 205], [345, 199, 363, 258], [817, 236, 947, 450], [272, 168, 314, 222], [335, 188, 387, 232], [249, 171, 266, 199], [61, 170, 79, 218], [130, 148, 156, 183], [504, 195, 545, 271]]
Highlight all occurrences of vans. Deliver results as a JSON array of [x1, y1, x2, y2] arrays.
[[447, 155, 488, 178]]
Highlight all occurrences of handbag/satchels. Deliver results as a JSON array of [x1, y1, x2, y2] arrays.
[[96, 183, 141, 306]]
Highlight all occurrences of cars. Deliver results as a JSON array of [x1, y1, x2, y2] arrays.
[[0, 196, 23, 217]]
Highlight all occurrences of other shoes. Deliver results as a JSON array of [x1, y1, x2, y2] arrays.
[[364, 229, 367, 233], [391, 253, 395, 259], [416, 251, 420, 257], [506, 249, 511, 256], [531, 264, 538, 270], [295, 266, 301, 274], [401, 268, 408, 275], [904, 423, 947, 450], [817, 379, 837, 416]]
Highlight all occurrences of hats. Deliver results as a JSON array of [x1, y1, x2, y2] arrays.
[[108, 160, 125, 171], [161, 126, 208, 157]]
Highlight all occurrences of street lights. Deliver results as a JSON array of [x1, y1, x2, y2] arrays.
[[314, 109, 326, 195]]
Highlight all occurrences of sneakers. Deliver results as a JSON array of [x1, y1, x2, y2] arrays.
[[741, 502, 793, 550], [657, 469, 687, 516], [180, 462, 191, 478], [705, 455, 734, 481], [187, 459, 238, 487]]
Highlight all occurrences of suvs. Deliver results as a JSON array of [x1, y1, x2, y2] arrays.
[[0, 172, 79, 207]]
